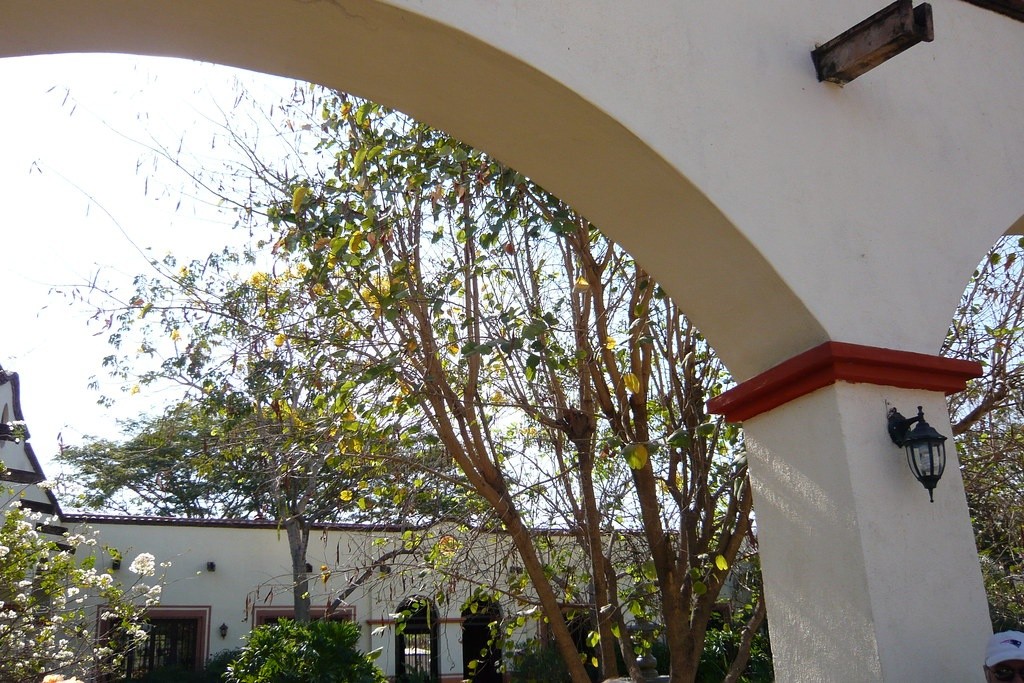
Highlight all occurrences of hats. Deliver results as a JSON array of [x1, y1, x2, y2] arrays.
[[985, 630, 1024, 666]]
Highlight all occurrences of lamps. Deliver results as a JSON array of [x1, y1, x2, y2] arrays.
[[887, 405, 948, 503], [219, 623, 228, 639]]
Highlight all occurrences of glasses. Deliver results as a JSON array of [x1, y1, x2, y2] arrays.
[[987, 666, 1024, 683]]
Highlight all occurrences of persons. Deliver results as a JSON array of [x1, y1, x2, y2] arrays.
[[983, 630, 1024, 683]]
